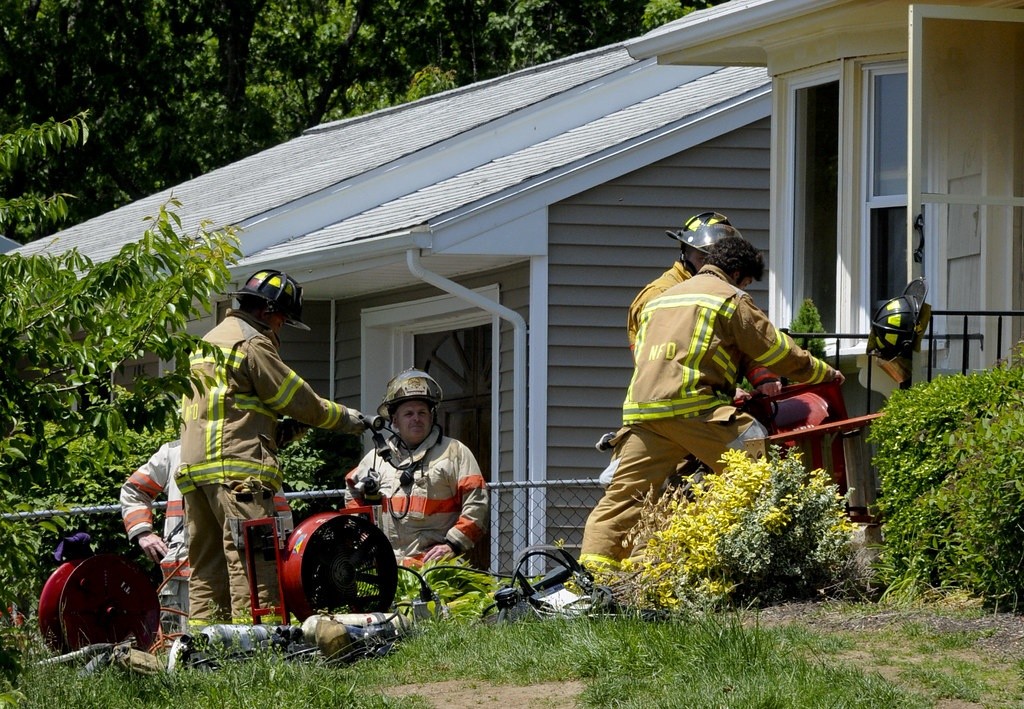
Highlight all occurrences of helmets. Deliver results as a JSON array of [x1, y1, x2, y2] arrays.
[[865, 280, 931, 384], [665, 211, 743, 255], [220, 268, 312, 331], [376, 366, 444, 422]]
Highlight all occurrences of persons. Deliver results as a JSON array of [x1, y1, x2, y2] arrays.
[[345, 366, 489, 567], [563, 211, 846, 598], [174, 268, 367, 627], [120, 439, 294, 655]]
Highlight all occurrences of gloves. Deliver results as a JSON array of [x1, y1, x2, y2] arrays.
[[342, 408, 369, 435]]
